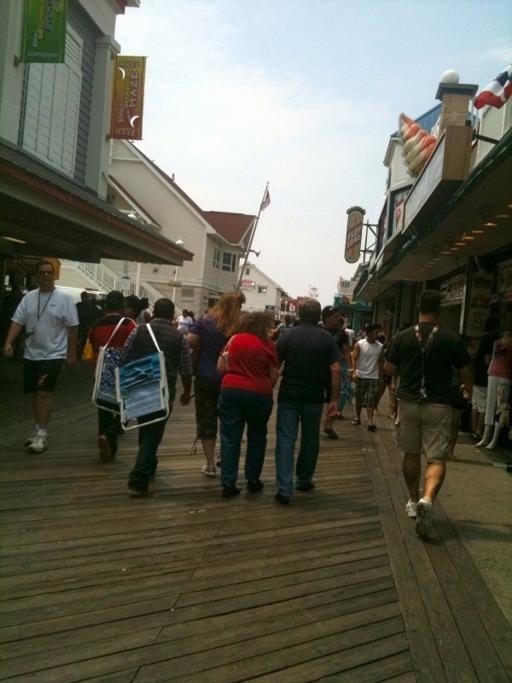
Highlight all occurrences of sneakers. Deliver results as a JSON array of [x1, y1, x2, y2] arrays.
[[24, 428, 39, 446], [275, 493, 290, 505], [99, 434, 118, 462], [295, 481, 314, 491], [201, 459, 221, 477], [414, 497, 433, 537], [404, 498, 417, 518], [27, 434, 48, 454], [324, 423, 338, 440], [222, 488, 240, 496], [246, 480, 265, 491], [125, 483, 150, 497]]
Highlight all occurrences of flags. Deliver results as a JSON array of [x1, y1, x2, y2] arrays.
[[260, 189, 270, 211]]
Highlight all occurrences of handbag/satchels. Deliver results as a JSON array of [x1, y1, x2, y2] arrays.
[[81, 342, 95, 366]]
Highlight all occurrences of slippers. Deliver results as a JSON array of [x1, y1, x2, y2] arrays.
[[352, 417, 360, 426], [366, 423, 376, 431]]
